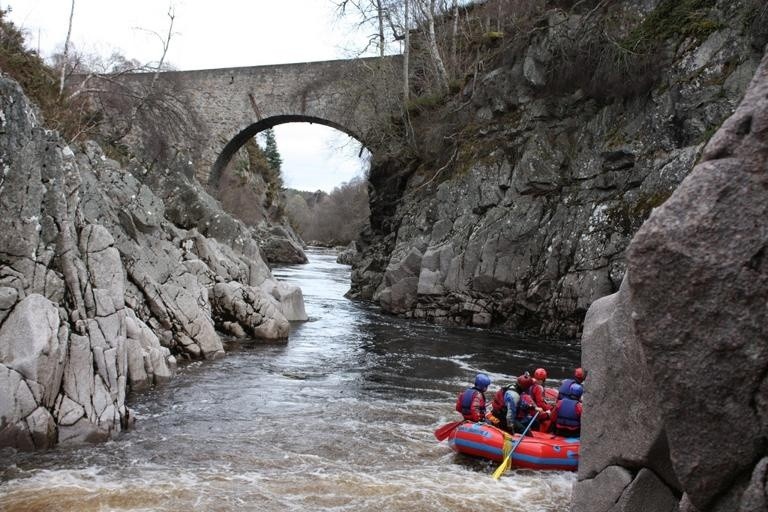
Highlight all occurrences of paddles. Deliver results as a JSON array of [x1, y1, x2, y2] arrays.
[[491, 411, 540, 479], [435, 398, 496, 441]]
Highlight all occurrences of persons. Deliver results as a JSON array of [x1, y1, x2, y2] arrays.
[[456, 367, 583, 437]]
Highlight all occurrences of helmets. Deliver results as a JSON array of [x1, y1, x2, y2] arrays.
[[476, 374, 490, 387], [518, 374, 533, 388], [570, 383, 583, 396], [534, 368, 547, 379], [575, 368, 582, 378]]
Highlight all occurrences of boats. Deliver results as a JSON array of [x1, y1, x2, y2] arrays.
[[455, 389, 579, 473]]
